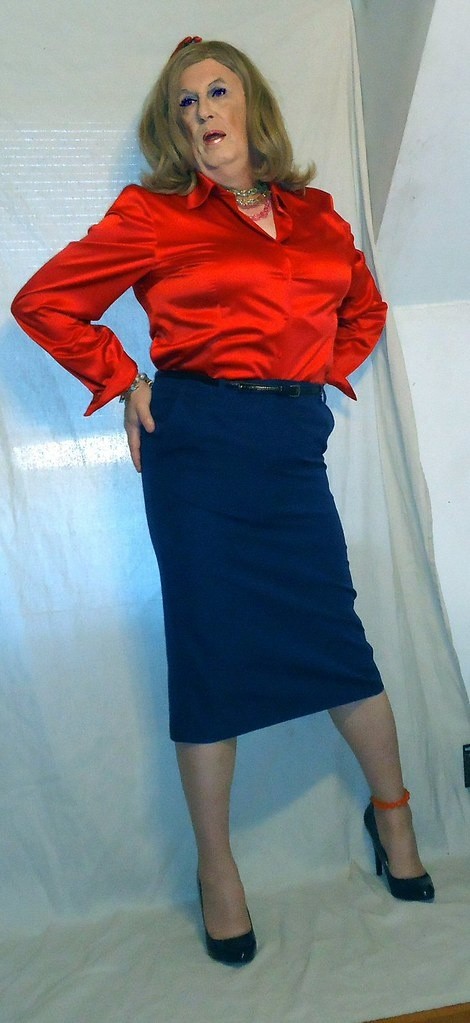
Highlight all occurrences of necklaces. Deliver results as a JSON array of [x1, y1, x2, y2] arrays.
[[226, 183, 270, 221]]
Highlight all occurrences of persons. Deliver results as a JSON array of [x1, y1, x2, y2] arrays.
[[9, 37, 435, 963]]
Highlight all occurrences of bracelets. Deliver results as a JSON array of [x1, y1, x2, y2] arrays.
[[119, 374, 153, 403]]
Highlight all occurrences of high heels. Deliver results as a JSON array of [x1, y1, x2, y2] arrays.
[[363, 804, 435, 901], [195, 867, 257, 964]]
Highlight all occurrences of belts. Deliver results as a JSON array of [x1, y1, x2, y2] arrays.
[[156, 371, 321, 397]]
